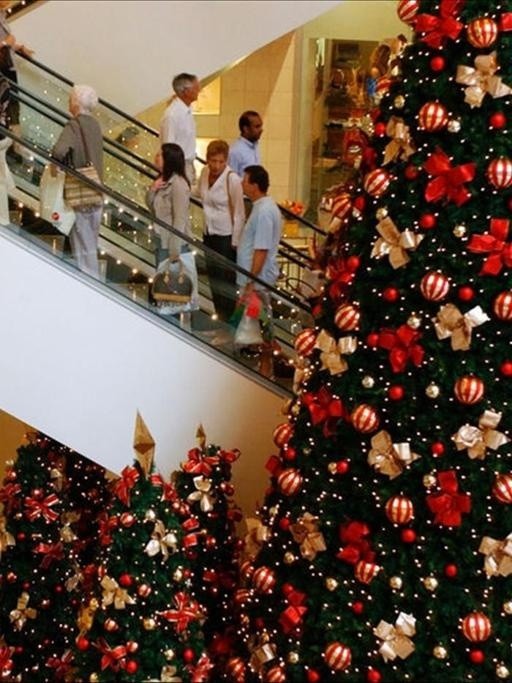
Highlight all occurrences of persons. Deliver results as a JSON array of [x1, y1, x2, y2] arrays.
[[226, 110, 264, 218], [158, 72, 201, 190], [145, 142, 190, 322], [0, 0, 35, 163], [51, 83, 105, 281], [190, 138, 246, 346], [236, 164, 282, 382], [369, 44, 391, 79]]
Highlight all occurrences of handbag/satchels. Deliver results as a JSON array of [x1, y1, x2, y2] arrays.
[[151, 257, 194, 304], [63, 160, 107, 210]]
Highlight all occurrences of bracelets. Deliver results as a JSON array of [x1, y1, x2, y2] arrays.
[[2, 39, 7, 46], [246, 278, 253, 285], [17, 42, 23, 51]]
